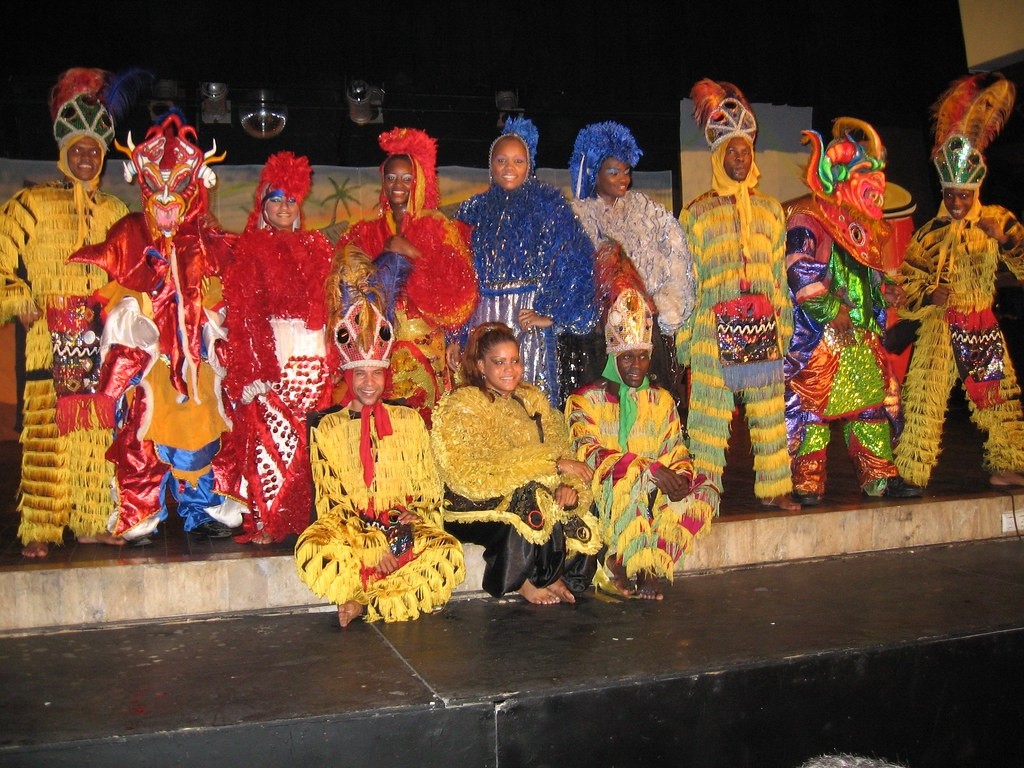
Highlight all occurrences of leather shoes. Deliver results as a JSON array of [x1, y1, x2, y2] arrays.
[[189, 521, 232, 538], [130, 538, 152, 546]]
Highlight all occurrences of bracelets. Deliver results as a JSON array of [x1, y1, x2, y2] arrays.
[[557, 459, 562, 474]]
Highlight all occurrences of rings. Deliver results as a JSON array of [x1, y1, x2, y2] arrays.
[[571, 464, 576, 469]]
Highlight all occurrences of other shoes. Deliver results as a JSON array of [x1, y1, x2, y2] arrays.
[[884, 475, 922, 498], [794, 492, 817, 506]]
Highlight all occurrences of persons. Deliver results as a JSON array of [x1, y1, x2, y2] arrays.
[[227, 149, 344, 544], [563, 288, 722, 601], [0, 66, 166, 556], [337, 127, 480, 431], [783, 117, 925, 507], [569, 122, 698, 429], [430, 322, 594, 605], [677, 78, 804, 511], [453, 115, 601, 408], [892, 131, 1024, 486], [65, 113, 233, 545], [294, 297, 465, 627]]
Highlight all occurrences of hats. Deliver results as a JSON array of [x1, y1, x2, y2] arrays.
[[933, 77, 1016, 191], [49, 66, 115, 151], [692, 80, 758, 150], [604, 287, 653, 359], [333, 251, 410, 370]]
